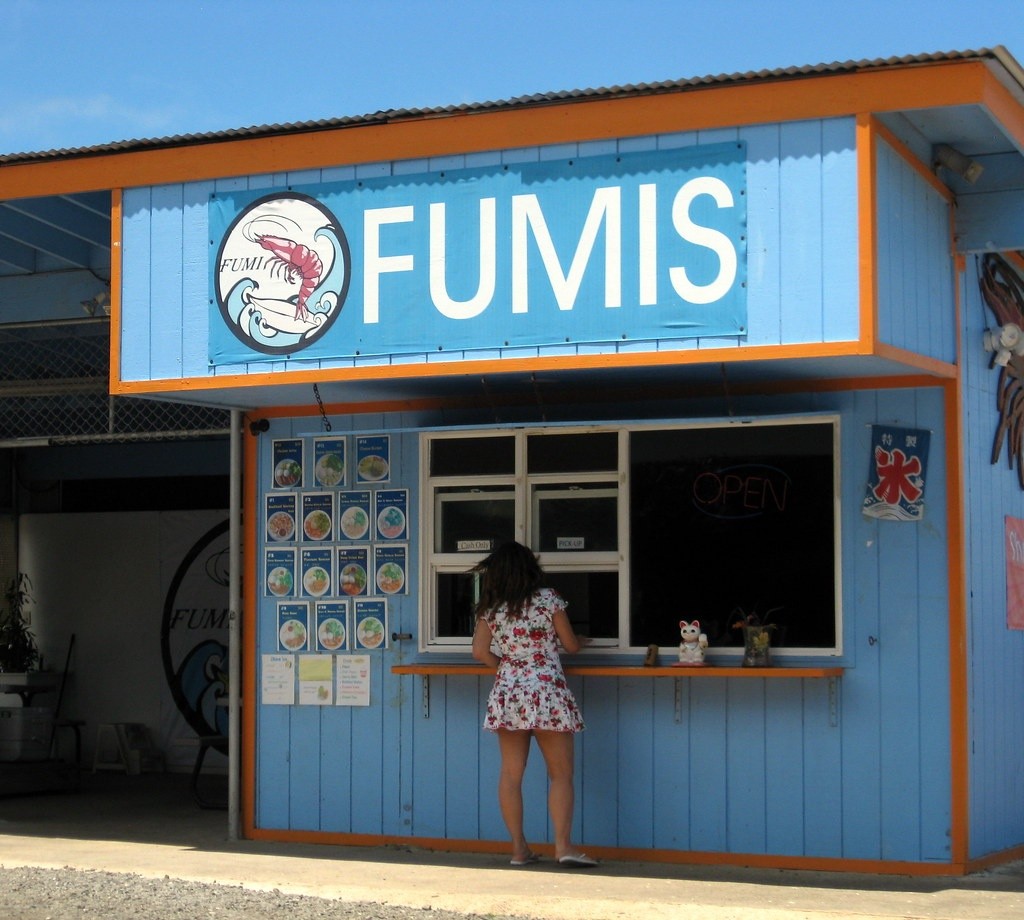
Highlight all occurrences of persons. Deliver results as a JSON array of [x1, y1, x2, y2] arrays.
[[472, 543, 599, 865]]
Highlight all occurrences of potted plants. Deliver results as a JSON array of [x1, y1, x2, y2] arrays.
[[0, 573, 50, 685]]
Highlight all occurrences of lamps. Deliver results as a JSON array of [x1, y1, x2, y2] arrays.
[[930, 142, 983, 186], [80, 290, 110, 316]]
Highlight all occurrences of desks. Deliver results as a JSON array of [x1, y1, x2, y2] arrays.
[[0, 672, 54, 707]]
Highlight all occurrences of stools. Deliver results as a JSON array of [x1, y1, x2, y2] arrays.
[[91, 723, 156, 778]]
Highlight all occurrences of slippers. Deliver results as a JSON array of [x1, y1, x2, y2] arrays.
[[558, 851, 598, 866], [510, 851, 538, 865]]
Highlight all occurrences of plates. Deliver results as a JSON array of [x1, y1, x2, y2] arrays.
[[303, 567, 330, 597], [318, 618, 346, 650], [358, 455, 389, 481], [275, 459, 301, 488], [304, 510, 331, 541], [377, 506, 406, 539], [267, 567, 293, 597], [280, 619, 306, 652], [341, 506, 369, 540], [356, 617, 385, 649], [315, 454, 345, 487], [377, 562, 405, 594], [340, 563, 366, 595], [267, 511, 294, 541]]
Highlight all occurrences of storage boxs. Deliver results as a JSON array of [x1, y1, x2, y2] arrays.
[[0, 706, 54, 762]]
